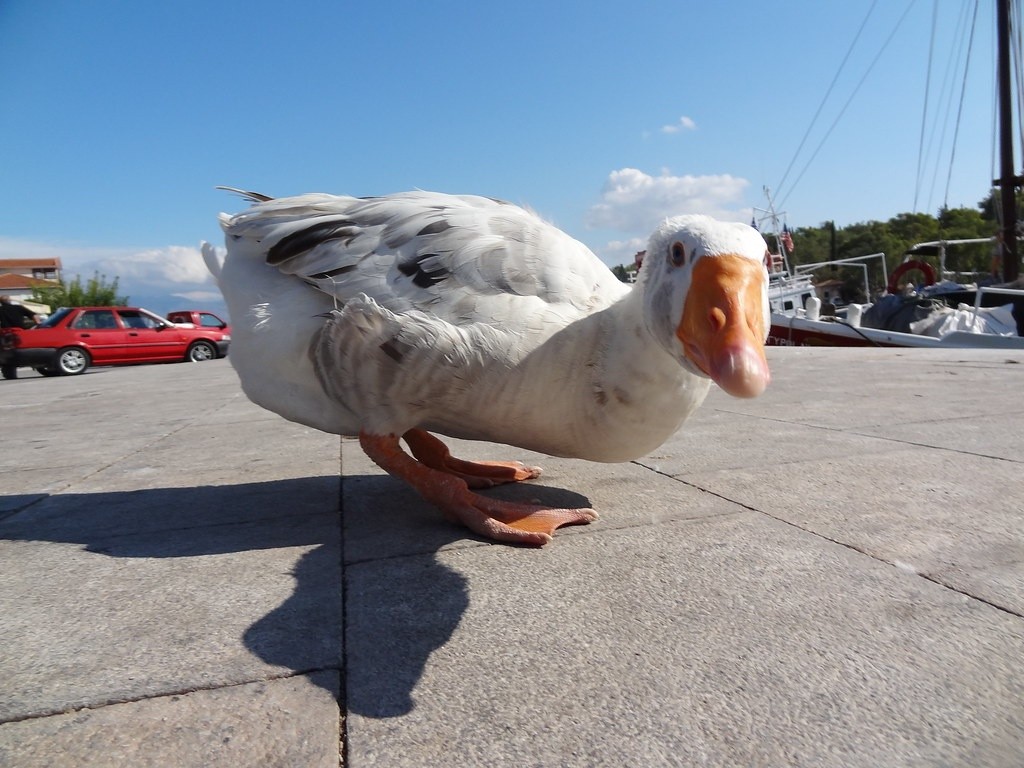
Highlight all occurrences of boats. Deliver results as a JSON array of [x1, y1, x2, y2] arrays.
[[626, 0, 1024, 349]]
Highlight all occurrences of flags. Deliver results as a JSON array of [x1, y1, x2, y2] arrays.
[[780, 223, 794, 252]]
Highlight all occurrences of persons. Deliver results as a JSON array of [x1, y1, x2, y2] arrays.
[[0, 295, 41, 379]]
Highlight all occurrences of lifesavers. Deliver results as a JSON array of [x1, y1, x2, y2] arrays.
[[889, 259, 936, 296]]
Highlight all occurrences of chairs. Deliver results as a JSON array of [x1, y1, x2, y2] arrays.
[[107, 316, 117, 327], [97, 318, 108, 328]]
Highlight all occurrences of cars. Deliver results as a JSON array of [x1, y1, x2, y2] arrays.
[[0, 307, 232, 375]]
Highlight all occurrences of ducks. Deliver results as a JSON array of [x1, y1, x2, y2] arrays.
[[199, 186, 772, 547]]
